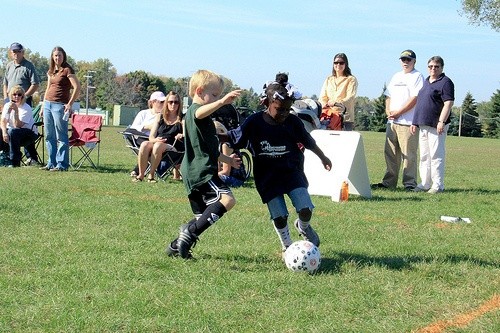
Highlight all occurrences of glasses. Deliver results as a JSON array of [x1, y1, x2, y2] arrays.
[[401, 58, 414, 62], [334, 61, 345, 66], [428, 65, 441, 68], [159, 102, 164, 103], [167, 101, 179, 105], [13, 93, 23, 97]]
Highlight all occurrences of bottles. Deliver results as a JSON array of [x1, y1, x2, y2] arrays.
[[342, 180, 349, 200], [6, 100, 15, 114], [62, 110, 70, 121]]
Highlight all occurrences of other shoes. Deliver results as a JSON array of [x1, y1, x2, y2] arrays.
[[371, 183, 386, 188], [428, 189, 443, 194], [405, 186, 415, 191], [40, 166, 53, 170], [415, 186, 429, 192], [51, 168, 63, 172]]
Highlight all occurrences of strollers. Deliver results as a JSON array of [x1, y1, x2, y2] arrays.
[[116, 127, 172, 179], [288, 99, 346, 153]]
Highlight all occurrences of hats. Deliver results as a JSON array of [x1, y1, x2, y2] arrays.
[[399, 50, 415, 59], [150, 92, 166, 102], [10, 43, 23, 51]]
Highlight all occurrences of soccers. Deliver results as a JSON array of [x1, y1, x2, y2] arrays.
[[285, 241, 323, 275]]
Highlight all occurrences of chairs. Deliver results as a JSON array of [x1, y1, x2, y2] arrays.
[[121, 125, 185, 182], [68, 114, 102, 171], [14, 101, 45, 168]]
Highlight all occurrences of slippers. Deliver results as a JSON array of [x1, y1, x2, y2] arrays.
[[133, 178, 142, 182], [148, 179, 156, 184]]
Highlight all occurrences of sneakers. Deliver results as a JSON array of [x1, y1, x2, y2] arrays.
[[167, 244, 193, 259], [294, 219, 320, 248], [177, 224, 199, 260]]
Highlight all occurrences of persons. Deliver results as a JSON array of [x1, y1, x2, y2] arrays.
[[133, 91, 185, 183], [219, 84, 332, 262], [132, 92, 166, 177], [2, 43, 40, 164], [410, 57, 454, 189], [40, 46, 80, 170], [372, 49, 424, 188], [209, 103, 248, 176], [0, 85, 38, 167], [319, 53, 357, 130], [166, 70, 242, 260]]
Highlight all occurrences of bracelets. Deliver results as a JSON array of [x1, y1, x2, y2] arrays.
[[438, 121, 446, 123]]
